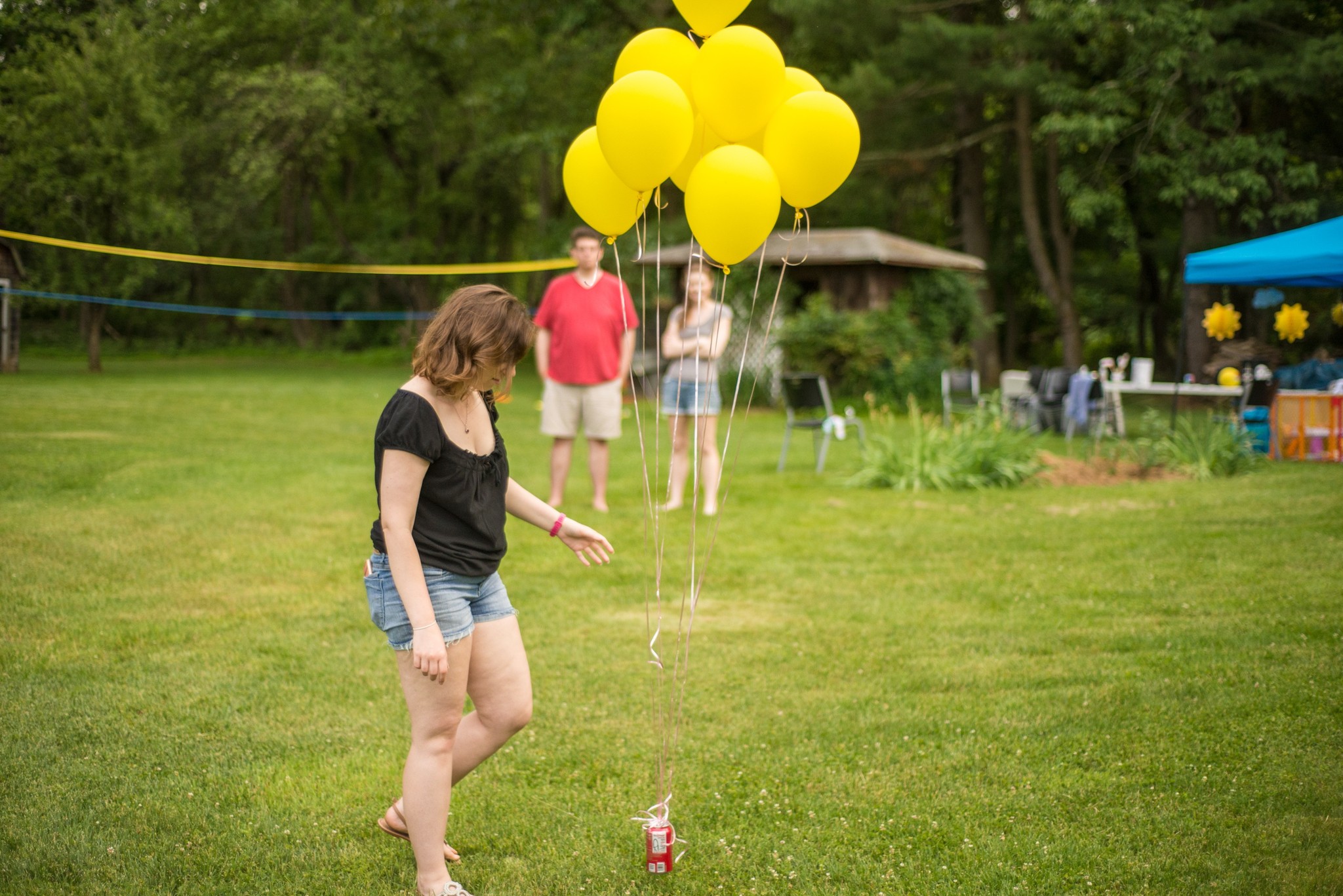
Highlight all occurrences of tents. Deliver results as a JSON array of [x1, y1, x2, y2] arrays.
[[1167, 214, 1343, 470]]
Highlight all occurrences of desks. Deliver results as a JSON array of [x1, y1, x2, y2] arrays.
[[1101, 381, 1244, 438]]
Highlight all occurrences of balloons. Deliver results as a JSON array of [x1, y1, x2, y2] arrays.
[[561, 0, 862, 269]]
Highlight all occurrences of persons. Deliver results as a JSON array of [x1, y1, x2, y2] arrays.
[[364, 284, 615, 896], [655, 261, 734, 516], [532, 226, 639, 513]]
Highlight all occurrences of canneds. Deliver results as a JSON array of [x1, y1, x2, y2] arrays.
[[646, 821, 673, 874]]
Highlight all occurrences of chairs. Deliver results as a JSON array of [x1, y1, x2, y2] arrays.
[[999, 367, 1103, 440], [776, 374, 865, 476], [940, 368, 980, 421]]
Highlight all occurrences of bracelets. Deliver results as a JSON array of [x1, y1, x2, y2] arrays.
[[412, 620, 436, 631], [549, 512, 566, 537]]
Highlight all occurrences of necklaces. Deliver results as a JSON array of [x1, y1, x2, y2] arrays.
[[449, 388, 470, 433]]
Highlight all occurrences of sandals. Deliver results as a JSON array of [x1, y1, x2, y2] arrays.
[[417, 881, 473, 896], [378, 804, 462, 865]]
